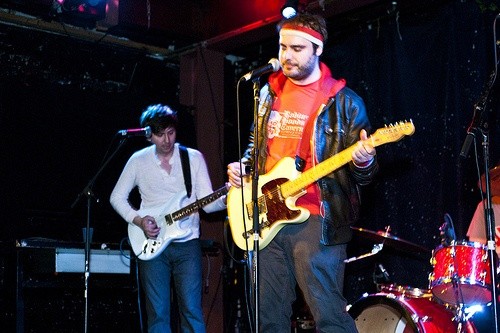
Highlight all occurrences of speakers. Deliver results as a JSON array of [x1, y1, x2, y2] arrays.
[[0, 239, 139, 333]]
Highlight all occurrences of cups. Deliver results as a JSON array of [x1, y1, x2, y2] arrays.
[[82, 228, 93, 243]]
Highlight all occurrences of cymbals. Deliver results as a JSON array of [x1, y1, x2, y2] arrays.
[[478, 165, 500, 196]]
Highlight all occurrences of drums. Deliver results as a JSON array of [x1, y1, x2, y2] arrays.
[[345, 283, 478, 333], [430, 240, 493, 305]]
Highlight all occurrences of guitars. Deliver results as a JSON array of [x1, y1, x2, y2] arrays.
[[227, 118, 416, 252], [128, 183, 230, 261]]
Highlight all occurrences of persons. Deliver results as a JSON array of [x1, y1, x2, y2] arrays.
[[227, 11, 378, 333], [108, 104, 233, 333], [466, 196, 500, 261]]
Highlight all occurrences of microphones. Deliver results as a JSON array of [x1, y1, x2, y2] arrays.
[[240, 57, 280, 82], [118, 125, 151, 137]]
